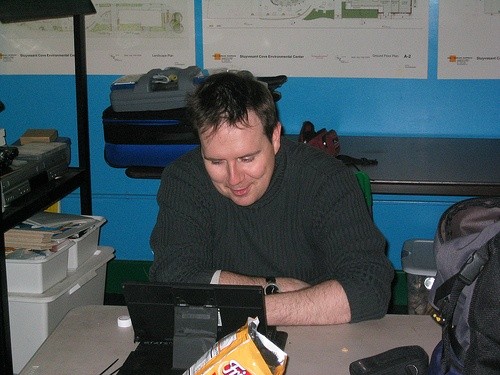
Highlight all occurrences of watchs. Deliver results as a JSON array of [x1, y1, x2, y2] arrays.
[[264, 277, 280, 295]]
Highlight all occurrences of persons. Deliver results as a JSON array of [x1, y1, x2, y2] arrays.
[[149, 72, 394, 327]]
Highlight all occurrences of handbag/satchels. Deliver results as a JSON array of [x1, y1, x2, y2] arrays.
[[298, 121, 341, 161]]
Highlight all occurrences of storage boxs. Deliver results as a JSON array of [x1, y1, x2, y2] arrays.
[[0, 137, 72, 214], [4, 242, 74, 295], [20, 129, 58, 144], [24, 211, 106, 268], [400, 237, 438, 314], [102, 76, 288, 168]]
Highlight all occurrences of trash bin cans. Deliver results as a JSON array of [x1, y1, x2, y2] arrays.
[[402, 240, 437, 315]]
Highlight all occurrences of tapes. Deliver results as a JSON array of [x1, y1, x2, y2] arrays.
[[118, 316, 132, 327]]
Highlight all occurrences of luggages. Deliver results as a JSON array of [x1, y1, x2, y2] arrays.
[[101, 105, 201, 169]]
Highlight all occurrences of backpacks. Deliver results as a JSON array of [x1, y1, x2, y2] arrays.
[[427, 197, 500, 374]]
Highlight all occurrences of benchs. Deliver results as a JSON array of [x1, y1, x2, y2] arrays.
[[283, 134, 499, 276]]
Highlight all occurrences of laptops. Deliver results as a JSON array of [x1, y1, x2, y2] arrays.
[[121, 281, 277, 370]]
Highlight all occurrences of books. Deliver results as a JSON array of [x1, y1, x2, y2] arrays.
[[3, 212, 100, 250]]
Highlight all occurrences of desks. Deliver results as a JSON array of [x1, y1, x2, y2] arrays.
[[18, 307, 442, 375]]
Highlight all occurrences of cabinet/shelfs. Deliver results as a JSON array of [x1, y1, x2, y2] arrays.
[[0, 0, 98, 375]]
